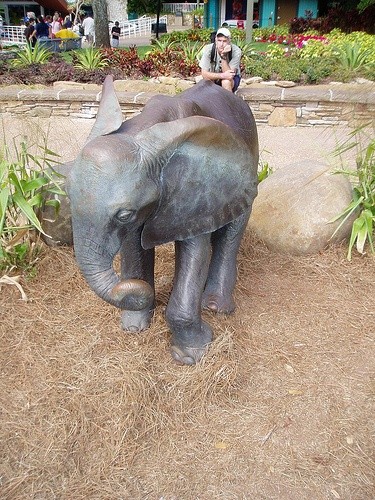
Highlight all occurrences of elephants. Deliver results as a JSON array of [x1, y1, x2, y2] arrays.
[[66, 74, 260, 364]]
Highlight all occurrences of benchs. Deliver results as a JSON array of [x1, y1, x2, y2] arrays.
[[36, 37, 83, 55]]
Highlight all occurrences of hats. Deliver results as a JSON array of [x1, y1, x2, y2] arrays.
[[217, 28, 231, 37]]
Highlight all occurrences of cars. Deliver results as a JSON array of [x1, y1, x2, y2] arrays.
[[223, 11, 260, 29]]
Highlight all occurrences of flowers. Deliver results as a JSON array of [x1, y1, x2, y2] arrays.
[[254, 35, 329, 57]]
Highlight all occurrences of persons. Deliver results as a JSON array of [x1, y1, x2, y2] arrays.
[[200, 27, 243, 93], [111, 21, 121, 48], [81, 12, 95, 45], [22, 10, 72, 53]]
